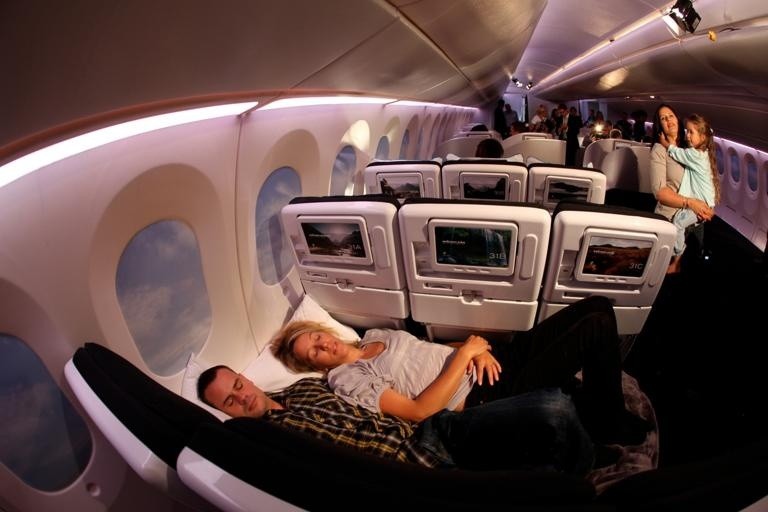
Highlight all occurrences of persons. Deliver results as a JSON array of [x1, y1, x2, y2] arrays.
[[658, 112, 720, 274], [195, 365, 601, 512], [649, 102, 704, 259], [453, 227, 488, 267], [269, 296, 651, 446], [465, 95, 648, 164]]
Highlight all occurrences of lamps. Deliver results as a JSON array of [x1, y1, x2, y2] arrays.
[[525, 82, 534, 90], [511, 77, 520, 85], [661, 0, 702, 42]]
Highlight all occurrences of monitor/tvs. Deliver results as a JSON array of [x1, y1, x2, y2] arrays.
[[543, 176, 593, 208], [460, 172, 510, 203], [296, 215, 373, 266], [614, 141, 632, 151], [523, 135, 547, 141], [467, 134, 492, 139], [376, 173, 424, 201], [428, 218, 519, 277], [574, 228, 658, 285]]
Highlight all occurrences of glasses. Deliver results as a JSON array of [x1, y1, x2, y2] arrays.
[[659, 114, 675, 122]]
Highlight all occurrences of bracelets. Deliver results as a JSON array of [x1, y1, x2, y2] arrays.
[[682, 196, 686, 210], [685, 197, 689, 210]]
